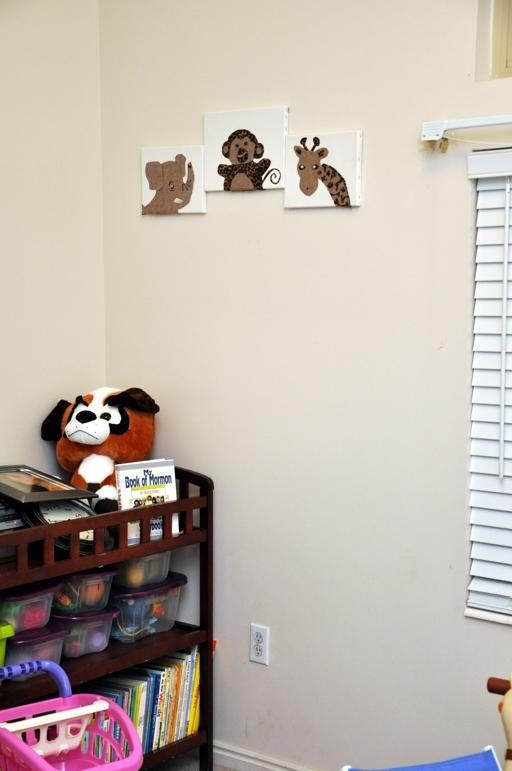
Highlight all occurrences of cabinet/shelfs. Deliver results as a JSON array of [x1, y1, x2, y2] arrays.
[[0, 467, 216, 769]]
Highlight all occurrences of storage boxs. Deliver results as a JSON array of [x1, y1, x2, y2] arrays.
[[0, 547, 187, 682]]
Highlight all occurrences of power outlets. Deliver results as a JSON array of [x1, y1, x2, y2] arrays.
[[248, 623, 270, 665]]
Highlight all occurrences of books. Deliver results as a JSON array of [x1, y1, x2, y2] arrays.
[[115, 457, 180, 542], [83, 646, 199, 758]]
